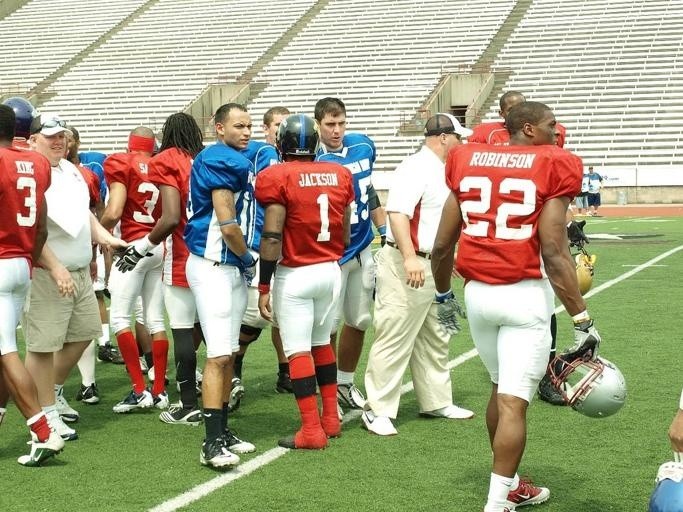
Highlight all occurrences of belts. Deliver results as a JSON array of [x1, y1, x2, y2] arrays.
[[386, 241, 433, 260]]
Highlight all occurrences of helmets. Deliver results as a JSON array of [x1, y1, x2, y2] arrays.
[[547, 353, 628, 418], [1, 97, 34, 137], [649, 461, 683, 512], [589, 166, 593, 169], [573, 252, 597, 297], [275, 114, 322, 156]]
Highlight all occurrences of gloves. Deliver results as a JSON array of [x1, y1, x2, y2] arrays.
[[381, 236, 386, 247], [567, 220, 589, 250]]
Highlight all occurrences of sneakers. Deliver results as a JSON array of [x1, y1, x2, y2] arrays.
[[227, 377, 245, 412], [506, 483, 551, 508], [418, 404, 474, 419], [319, 415, 341, 438], [199, 441, 241, 468], [335, 383, 368, 409], [76, 383, 100, 403], [220, 434, 256, 454], [148, 365, 170, 386], [275, 372, 296, 393], [146, 387, 171, 409], [138, 357, 149, 374], [96, 341, 125, 364], [196, 370, 204, 384], [159, 405, 204, 427], [176, 379, 202, 397], [50, 416, 78, 441], [360, 410, 399, 436], [55, 395, 79, 422], [277, 430, 328, 449], [17, 431, 66, 467], [112, 390, 155, 413]]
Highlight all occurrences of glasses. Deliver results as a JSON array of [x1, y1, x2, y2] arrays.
[[41, 120, 67, 127]]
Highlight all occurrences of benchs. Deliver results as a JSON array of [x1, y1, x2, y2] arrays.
[[0, 0, 157, 97], [480, 0, 682, 165]]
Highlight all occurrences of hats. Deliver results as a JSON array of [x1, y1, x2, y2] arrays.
[[29, 112, 73, 138], [424, 112, 474, 138]]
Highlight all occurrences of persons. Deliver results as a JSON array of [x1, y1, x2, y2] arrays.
[[575, 171, 591, 218], [1, 95, 391, 465], [466, 88, 573, 407], [585, 165, 602, 216], [430, 100, 602, 512], [360, 112, 474, 436], [667, 388, 683, 457]]
